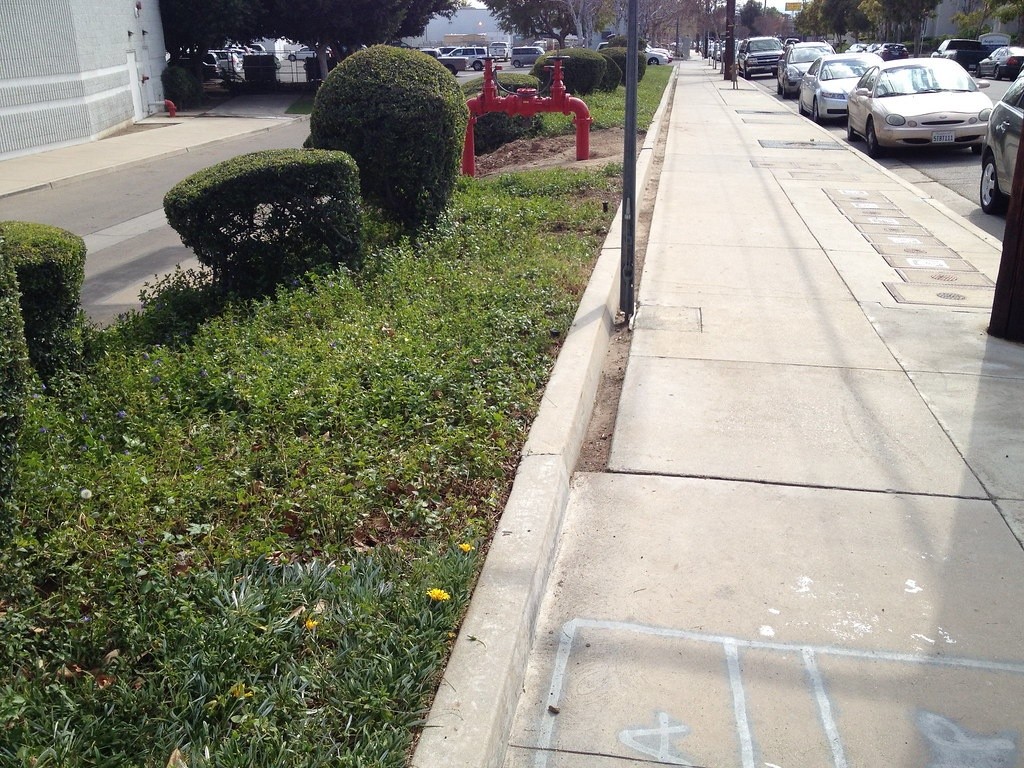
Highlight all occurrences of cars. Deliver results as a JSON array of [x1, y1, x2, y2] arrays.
[[799, 53, 887, 126], [863, 43, 881, 53], [980, 70, 1024, 216], [847, 58, 994, 159], [166, 44, 267, 81], [844, 44, 870, 53], [975, 46, 1024, 81], [699, 39, 744, 69]]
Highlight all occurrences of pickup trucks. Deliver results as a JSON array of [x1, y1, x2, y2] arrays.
[[419, 49, 470, 76], [930, 39, 992, 72], [489, 42, 513, 62]]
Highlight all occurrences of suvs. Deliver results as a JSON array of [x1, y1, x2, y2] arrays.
[[287, 45, 332, 62], [737, 36, 786, 80], [437, 46, 456, 55], [873, 43, 909, 61], [443, 47, 493, 71], [783, 38, 800, 51], [595, 42, 668, 66], [647, 43, 673, 63], [777, 39, 836, 99]]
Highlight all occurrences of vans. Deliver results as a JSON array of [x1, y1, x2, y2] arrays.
[[532, 41, 548, 51], [511, 47, 545, 68]]
